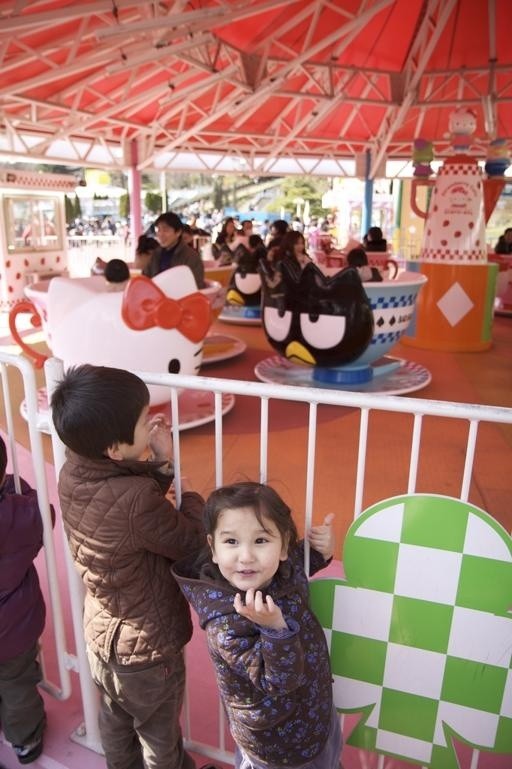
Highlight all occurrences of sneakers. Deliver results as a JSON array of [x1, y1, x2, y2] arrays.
[[12, 713, 47, 764]]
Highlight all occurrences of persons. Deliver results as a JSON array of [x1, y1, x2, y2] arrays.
[[0, 438, 55, 763], [169, 483, 342, 769], [51, 364, 205, 769], [495, 228, 512, 254], [106, 212, 387, 289]]
